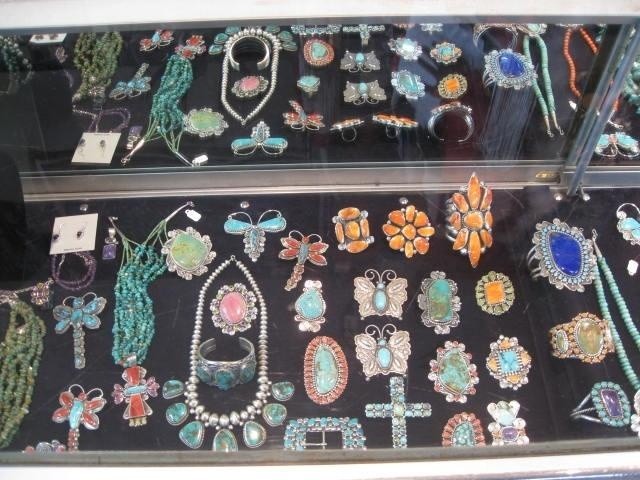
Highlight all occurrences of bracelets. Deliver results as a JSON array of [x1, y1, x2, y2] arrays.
[[481, 47, 538, 90], [426, 101, 475, 144], [526, 219, 598, 294], [197, 335, 256, 390], [229, 34, 271, 71], [472, 22, 518, 51], [571, 381, 631, 427], [548, 313, 616, 364]]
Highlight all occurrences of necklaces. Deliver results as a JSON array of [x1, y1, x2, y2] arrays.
[[558, 23, 624, 129], [163, 254, 295, 453], [588, 229, 640, 443], [209, 24, 298, 126], [516, 23, 564, 138]]
